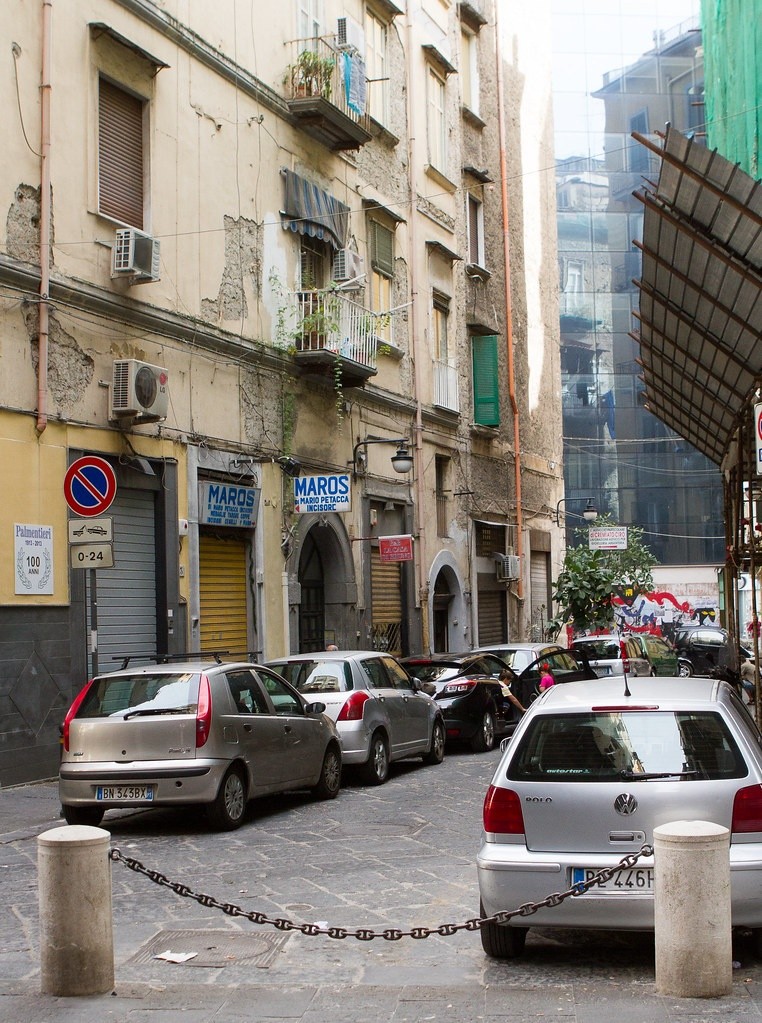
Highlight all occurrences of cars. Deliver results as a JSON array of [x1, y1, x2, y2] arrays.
[[395, 650, 600, 754], [246, 649, 447, 785], [467, 642, 582, 678], [56, 648, 344, 833], [566, 624, 755, 679], [472, 675, 762, 963]]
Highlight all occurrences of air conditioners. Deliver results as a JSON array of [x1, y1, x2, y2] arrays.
[[501, 555, 521, 578], [114, 229, 161, 279], [112, 358, 169, 417]]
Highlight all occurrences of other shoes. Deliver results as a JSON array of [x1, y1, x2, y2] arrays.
[[747, 700, 755, 705]]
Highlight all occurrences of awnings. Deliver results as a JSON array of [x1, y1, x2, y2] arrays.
[[471, 517, 518, 527]]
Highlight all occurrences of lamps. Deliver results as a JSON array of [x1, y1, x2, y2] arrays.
[[353, 437, 413, 484], [552, 496, 598, 527], [228, 456, 303, 478], [122, 456, 155, 476]]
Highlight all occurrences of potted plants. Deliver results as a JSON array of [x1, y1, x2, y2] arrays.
[[270, 270, 346, 454], [284, 49, 333, 99]]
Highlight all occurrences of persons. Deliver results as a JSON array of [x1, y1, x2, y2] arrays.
[[537, 663, 556, 692], [326, 645, 338, 651], [739, 654, 762, 705], [498, 669, 529, 712]]
